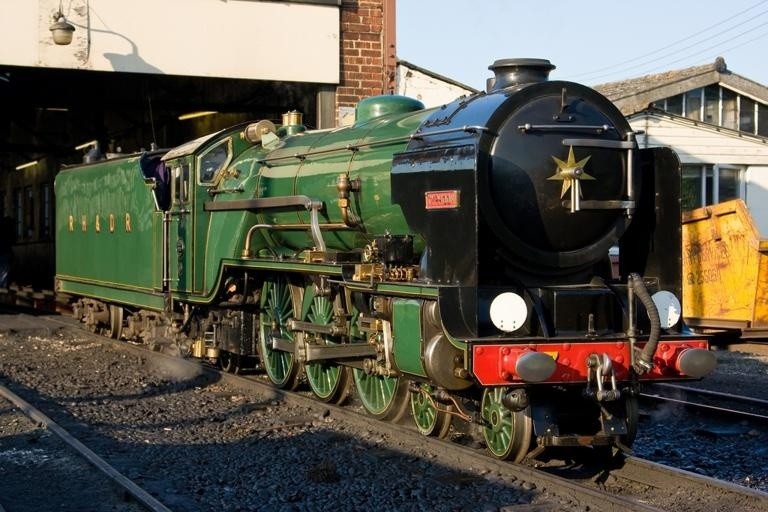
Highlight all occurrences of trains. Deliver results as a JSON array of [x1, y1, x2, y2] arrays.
[[1, 58, 715, 475]]
